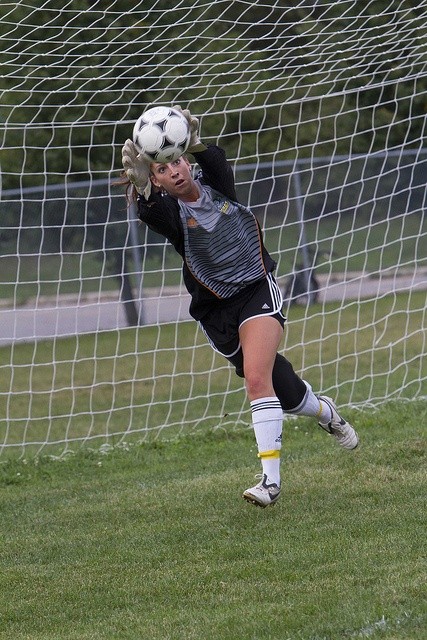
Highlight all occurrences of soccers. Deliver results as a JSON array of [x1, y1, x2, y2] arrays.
[[133, 106, 191, 163]]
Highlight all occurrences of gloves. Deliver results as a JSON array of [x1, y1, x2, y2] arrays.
[[121, 139, 153, 200], [172, 105, 207, 154]]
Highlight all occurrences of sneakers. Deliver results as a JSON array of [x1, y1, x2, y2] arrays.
[[317, 396, 359, 450], [242, 473, 282, 509]]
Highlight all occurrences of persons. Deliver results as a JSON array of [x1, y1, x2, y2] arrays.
[[120, 105, 359, 506]]
